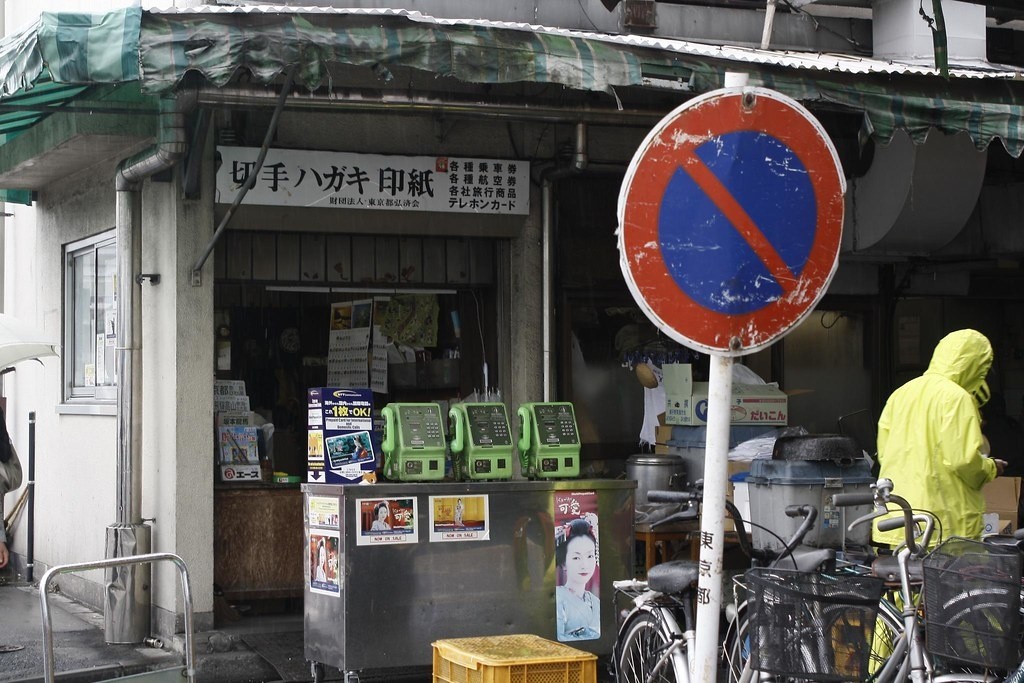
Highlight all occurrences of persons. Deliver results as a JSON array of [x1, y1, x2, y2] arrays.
[[554, 511, 600, 642], [613, 324, 695, 455], [315, 538, 327, 582], [866, 328, 1007, 683], [0, 404, 22, 569], [454, 499, 465, 527], [370, 502, 392, 530]]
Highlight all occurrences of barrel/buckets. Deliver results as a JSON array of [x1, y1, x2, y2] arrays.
[[625, 453, 687, 524]]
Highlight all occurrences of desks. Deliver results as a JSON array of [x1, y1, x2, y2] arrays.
[[634, 519, 700, 571], [690, 529, 751, 622]]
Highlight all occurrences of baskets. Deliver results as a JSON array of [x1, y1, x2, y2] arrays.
[[744, 567, 885, 682], [921, 535, 1024, 672]]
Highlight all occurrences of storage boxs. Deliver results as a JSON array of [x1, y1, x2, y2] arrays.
[[660, 363, 789, 427], [981, 476, 1021, 535], [654, 384, 814, 453], [306, 387, 378, 485], [221, 464, 261, 481], [430, 634, 597, 683], [750, 457, 877, 551], [726, 462, 752, 517]]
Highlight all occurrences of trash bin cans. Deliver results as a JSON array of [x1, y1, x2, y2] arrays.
[[744, 458, 877, 571]]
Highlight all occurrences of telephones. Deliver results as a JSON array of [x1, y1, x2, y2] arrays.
[[380, 401, 447, 482], [518, 402, 580, 478], [449, 402, 513, 482]]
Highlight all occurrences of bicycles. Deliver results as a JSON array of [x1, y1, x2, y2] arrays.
[[608, 478, 1024, 683]]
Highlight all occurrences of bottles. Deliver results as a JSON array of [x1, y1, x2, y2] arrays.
[[260, 455, 277, 486]]
